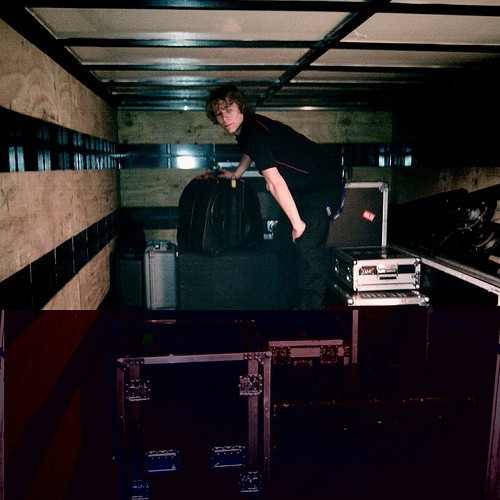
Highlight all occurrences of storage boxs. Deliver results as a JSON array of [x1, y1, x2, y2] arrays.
[[113, 351, 271, 500], [253, 338, 350, 369], [332, 247, 423, 292]]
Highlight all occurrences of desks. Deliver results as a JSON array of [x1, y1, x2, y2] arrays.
[[271, 391, 479, 450]]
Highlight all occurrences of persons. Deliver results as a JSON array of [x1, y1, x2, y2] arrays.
[[205, 83, 346, 312]]
